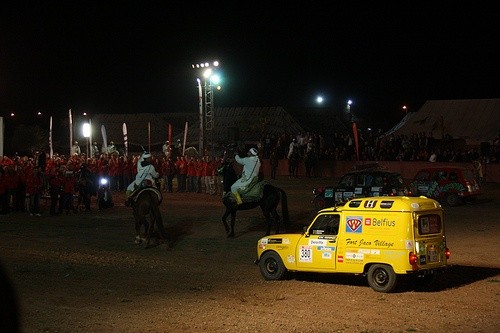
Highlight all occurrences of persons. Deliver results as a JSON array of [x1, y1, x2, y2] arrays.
[[262, 130, 435, 178], [107, 141, 119, 155], [70, 140, 81, 156], [0, 152, 226, 218], [125, 152, 160, 202], [231, 148, 261, 208], [92, 141, 99, 154], [162, 140, 171, 161]]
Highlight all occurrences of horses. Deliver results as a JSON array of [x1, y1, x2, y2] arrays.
[[213, 160, 290, 236], [126, 187, 165, 247]]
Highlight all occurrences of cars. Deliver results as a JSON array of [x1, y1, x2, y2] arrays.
[[311, 163, 413, 215], [404, 167, 484, 207]]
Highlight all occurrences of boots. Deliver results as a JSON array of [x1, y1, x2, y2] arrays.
[[232, 192, 243, 205]]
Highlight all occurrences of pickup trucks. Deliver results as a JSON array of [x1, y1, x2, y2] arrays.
[[253, 192, 450, 294]]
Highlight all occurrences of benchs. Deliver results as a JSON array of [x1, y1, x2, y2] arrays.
[[40, 195, 79, 210]]
